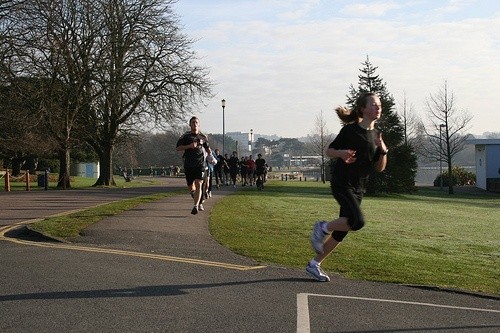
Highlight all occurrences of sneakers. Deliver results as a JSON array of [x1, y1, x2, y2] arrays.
[[305, 262, 330, 282], [192, 205, 198, 215], [311, 221, 330, 254], [202, 191, 212, 203], [199, 204, 204, 211]]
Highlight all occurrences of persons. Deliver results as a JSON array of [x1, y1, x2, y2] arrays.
[[137, 165, 182, 178], [304, 93, 389, 283], [175, 117, 269, 217]]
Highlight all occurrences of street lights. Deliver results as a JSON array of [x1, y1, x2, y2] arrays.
[[221, 99, 226, 157]]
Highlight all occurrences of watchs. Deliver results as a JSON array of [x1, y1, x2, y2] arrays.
[[379, 149, 389, 156]]
